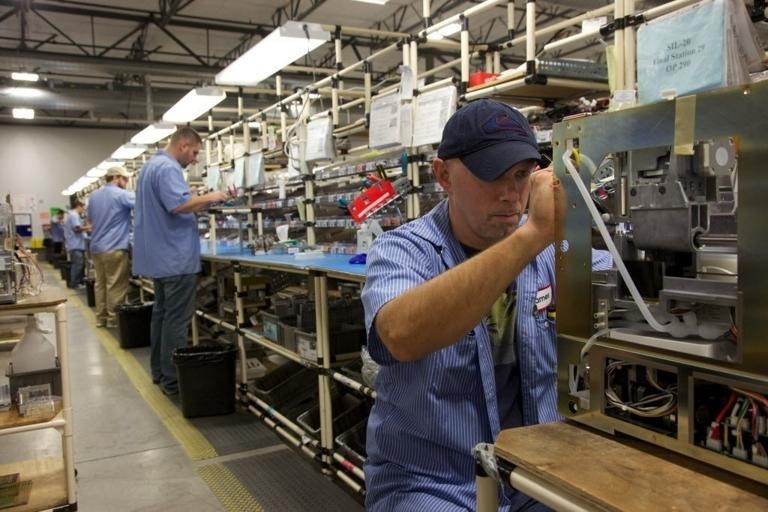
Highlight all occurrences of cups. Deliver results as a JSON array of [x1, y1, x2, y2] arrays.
[[581, 19, 601, 34], [611, 89, 634, 109], [253, 232, 275, 252]]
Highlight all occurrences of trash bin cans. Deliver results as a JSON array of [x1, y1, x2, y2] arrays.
[[171, 344, 239, 418], [54, 253, 72, 280], [113, 301, 154, 349], [82, 276, 96, 307]]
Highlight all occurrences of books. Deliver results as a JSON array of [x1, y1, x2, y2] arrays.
[[233, 85, 457, 188]]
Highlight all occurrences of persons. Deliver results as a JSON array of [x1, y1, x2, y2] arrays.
[[360, 99, 612, 512], [64, 200, 92, 289], [133, 126, 228, 394], [88, 166, 137, 328], [50, 210, 65, 254]]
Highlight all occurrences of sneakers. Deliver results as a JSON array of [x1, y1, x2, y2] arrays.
[[72, 283, 87, 290], [161, 386, 178, 396], [152, 377, 161, 384], [106, 319, 119, 329], [95, 317, 108, 328]]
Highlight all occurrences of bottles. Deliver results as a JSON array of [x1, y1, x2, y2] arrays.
[[355, 223, 372, 255], [278, 173, 286, 200]]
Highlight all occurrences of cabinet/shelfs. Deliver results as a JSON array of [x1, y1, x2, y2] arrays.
[[128, 239, 369, 502], [0, 285, 81, 512]]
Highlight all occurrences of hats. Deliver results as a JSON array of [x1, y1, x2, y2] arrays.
[[103, 164, 135, 178], [436, 97, 543, 182]]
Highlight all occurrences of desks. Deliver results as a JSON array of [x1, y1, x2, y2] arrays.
[[468, 422, 768, 512]]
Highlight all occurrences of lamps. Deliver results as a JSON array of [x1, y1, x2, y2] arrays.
[[96, 16, 336, 171]]
